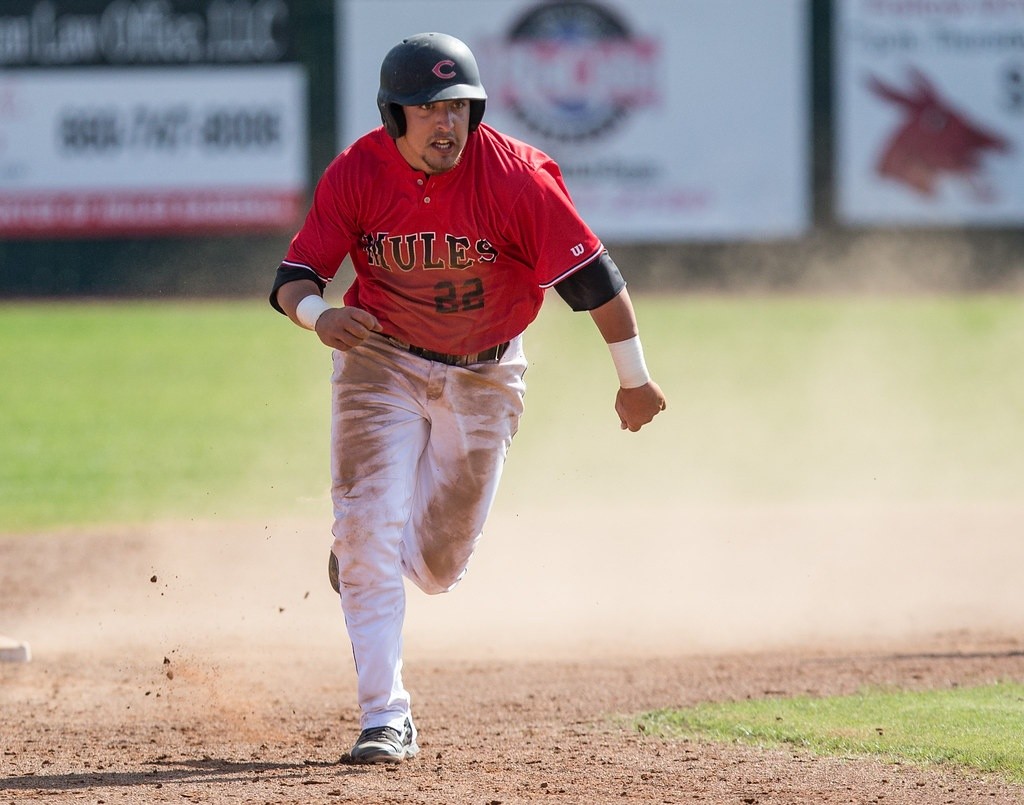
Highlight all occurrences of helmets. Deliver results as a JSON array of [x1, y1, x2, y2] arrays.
[[377, 33, 487, 137]]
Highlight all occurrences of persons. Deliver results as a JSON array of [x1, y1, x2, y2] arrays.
[[267, 31, 666, 762]]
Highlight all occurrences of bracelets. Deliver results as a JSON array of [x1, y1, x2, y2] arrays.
[[295, 295, 334, 332], [608, 334, 650, 388]]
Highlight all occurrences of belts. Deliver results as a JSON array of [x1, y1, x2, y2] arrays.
[[378, 334, 510, 367]]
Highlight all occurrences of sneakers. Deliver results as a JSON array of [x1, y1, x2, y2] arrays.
[[329, 550, 338, 594], [351, 716, 419, 762]]
